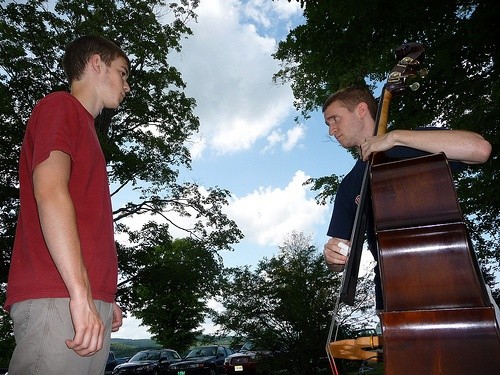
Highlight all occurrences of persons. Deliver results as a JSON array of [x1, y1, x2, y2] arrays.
[[323, 86, 491, 336], [5, 34, 130, 375]]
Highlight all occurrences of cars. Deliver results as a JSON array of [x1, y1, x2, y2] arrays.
[[104, 352, 130, 375], [112, 349, 182, 375], [224, 338, 289, 375], [169, 346, 236, 375]]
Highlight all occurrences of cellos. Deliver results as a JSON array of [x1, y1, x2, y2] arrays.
[[324, 42, 500, 375]]
